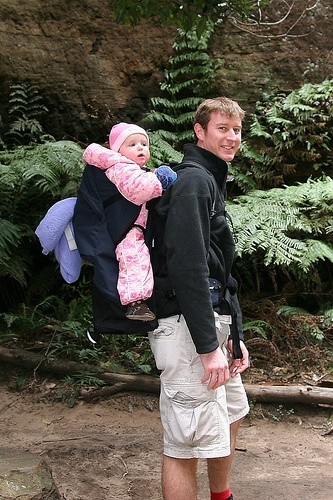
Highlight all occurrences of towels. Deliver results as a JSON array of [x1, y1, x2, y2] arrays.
[[35, 196, 83, 283]]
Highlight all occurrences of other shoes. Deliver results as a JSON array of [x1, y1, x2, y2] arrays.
[[124, 304, 156, 320]]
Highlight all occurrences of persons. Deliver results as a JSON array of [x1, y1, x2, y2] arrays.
[[106, 122, 178, 320], [146, 97, 253, 500]]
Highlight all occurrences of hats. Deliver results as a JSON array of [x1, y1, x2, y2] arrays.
[[107, 121, 148, 152]]
